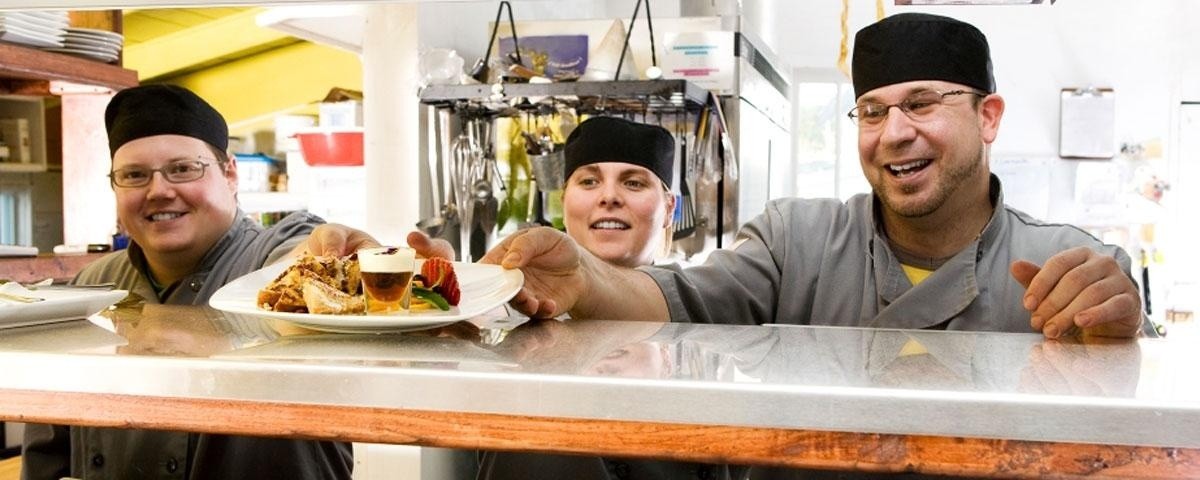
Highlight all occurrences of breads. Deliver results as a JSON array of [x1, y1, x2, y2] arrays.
[[257, 253, 365, 314]]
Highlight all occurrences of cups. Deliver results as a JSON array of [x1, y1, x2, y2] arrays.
[[358, 247, 416, 315]]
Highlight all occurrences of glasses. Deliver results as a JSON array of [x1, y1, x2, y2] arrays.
[[104, 160, 229, 187], [847, 90, 985, 130]]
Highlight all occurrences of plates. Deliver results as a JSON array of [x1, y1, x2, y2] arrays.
[[206, 335, 523, 380], [208, 255, 526, 333], [0, 9, 69, 53], [2, 318, 127, 354], [0, 281, 129, 331], [70, 30, 124, 65]]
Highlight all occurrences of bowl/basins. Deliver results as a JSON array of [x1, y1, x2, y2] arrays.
[[293, 128, 367, 168]]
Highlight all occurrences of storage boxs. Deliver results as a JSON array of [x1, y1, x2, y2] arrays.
[[318, 99, 362, 130], [237, 156, 273, 192]]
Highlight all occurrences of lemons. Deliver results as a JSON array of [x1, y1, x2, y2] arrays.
[[413, 285, 451, 312]]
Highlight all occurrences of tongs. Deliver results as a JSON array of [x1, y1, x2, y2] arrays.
[[685, 89, 738, 183]]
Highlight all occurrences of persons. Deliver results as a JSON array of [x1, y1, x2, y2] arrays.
[[405, 113, 736, 480], [19, 82, 387, 479], [478, 7, 1148, 342]]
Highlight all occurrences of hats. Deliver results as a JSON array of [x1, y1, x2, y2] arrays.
[[563, 116, 677, 190], [104, 83, 230, 157], [851, 12, 998, 99]]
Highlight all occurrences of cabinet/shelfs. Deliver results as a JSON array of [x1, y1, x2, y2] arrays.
[[0, 42, 139, 287]]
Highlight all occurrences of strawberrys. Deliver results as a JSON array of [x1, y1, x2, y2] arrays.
[[421, 257, 460, 305]]
